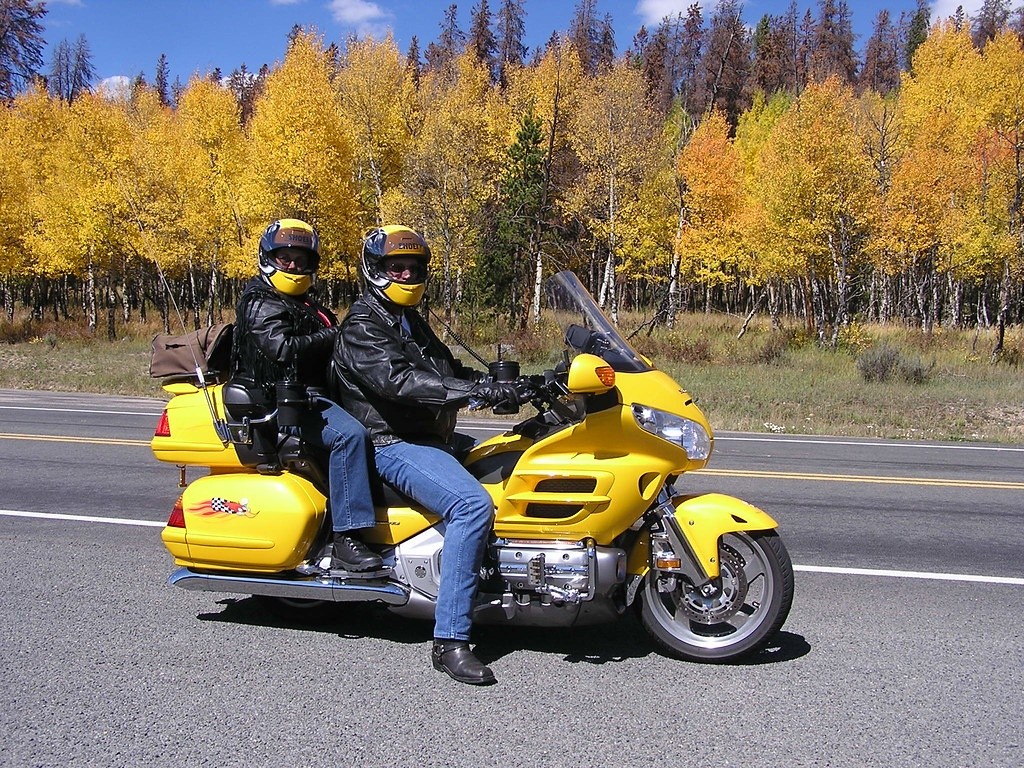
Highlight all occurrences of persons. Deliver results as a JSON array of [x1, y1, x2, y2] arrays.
[[327, 225, 567, 685], [229, 219, 386, 572]]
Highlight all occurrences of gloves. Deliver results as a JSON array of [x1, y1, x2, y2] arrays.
[[471, 382, 522, 406]]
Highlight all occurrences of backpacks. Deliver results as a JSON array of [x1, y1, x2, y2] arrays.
[[300, 302, 340, 387]]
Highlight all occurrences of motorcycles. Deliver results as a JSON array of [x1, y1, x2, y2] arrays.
[[149, 269, 797, 664]]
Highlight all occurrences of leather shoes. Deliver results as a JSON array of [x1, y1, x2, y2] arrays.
[[432, 638, 495, 684], [330, 534, 383, 572]]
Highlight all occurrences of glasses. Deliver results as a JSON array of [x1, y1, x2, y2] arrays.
[[276, 253, 307, 265], [386, 263, 424, 273]]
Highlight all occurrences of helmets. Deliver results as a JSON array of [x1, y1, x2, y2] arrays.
[[258, 219, 320, 296], [361, 224, 431, 307]]
